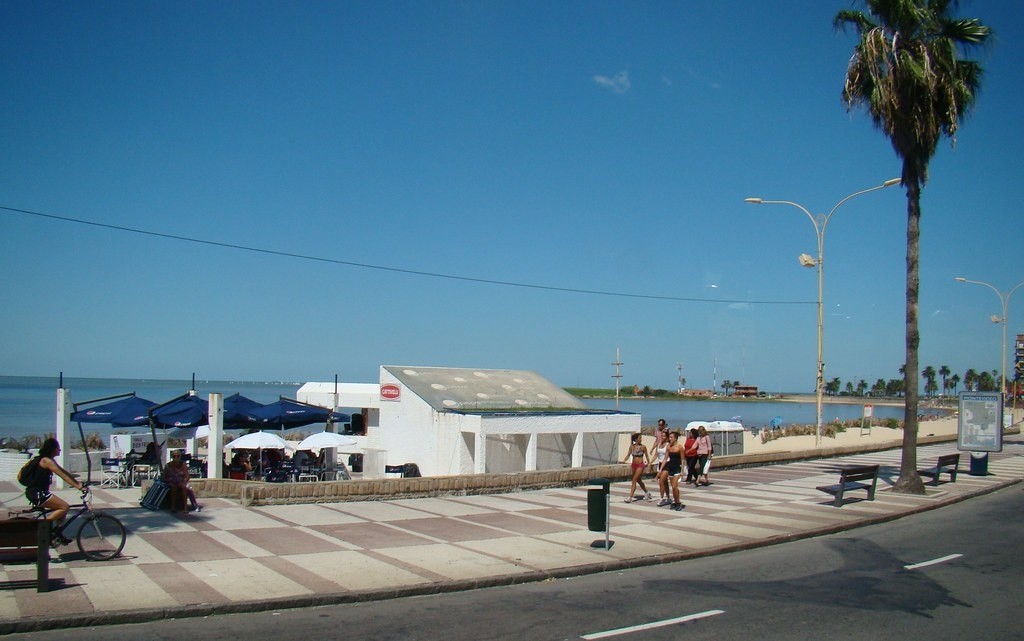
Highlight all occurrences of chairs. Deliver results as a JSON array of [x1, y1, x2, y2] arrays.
[[101, 457, 128, 489], [234, 460, 345, 481], [132, 456, 163, 490]]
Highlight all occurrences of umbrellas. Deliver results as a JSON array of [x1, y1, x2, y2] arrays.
[[224, 430, 285, 476], [771, 416, 782, 425], [68, 391, 350, 483], [295, 430, 356, 470]]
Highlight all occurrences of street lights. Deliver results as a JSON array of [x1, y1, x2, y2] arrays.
[[742, 178, 904, 449], [954, 277, 1024, 411]]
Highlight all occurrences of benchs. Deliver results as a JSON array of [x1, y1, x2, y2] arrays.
[[915, 452, 961, 486], [0, 517, 54, 593], [817, 463, 880, 506]]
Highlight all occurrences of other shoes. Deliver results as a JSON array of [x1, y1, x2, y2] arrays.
[[676, 503, 682, 511], [686, 479, 692, 483], [702, 482, 708, 486], [624, 497, 632, 502], [693, 482, 698, 487], [643, 492, 651, 499], [657, 499, 665, 506], [670, 504, 676, 508], [53, 527, 73, 544], [665, 499, 672, 504]]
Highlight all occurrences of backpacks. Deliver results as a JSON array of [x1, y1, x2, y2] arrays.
[[18, 456, 42, 486]]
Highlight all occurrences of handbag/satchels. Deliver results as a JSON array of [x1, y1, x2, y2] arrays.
[[703, 460, 711, 475], [705, 435, 714, 455]]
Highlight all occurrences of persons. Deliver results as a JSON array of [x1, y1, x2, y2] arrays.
[[161, 450, 189, 513], [26, 437, 84, 545], [623, 433, 651, 502], [682, 428, 698, 484], [649, 419, 684, 510], [685, 426, 712, 487]]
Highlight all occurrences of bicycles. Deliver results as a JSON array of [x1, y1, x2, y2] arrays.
[[6, 481, 128, 561]]
